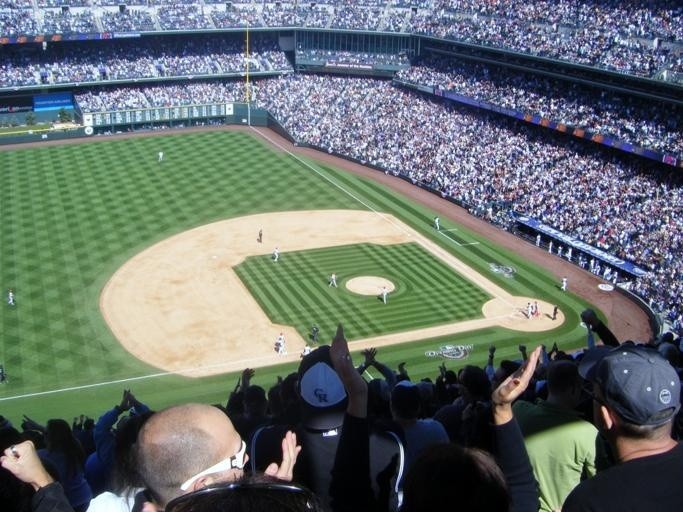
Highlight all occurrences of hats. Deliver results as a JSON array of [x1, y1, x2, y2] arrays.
[[296, 343, 350, 431], [577, 342, 683, 427]]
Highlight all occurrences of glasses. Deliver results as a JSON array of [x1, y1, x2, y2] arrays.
[[178, 438, 248, 493], [162, 479, 316, 512]]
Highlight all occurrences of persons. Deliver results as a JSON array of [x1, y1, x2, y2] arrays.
[[271, 247, 279, 262], [328, 271, 338, 289], [256, 229, 262, 243], [6, 288, 14, 305], [378, 285, 388, 306]]
